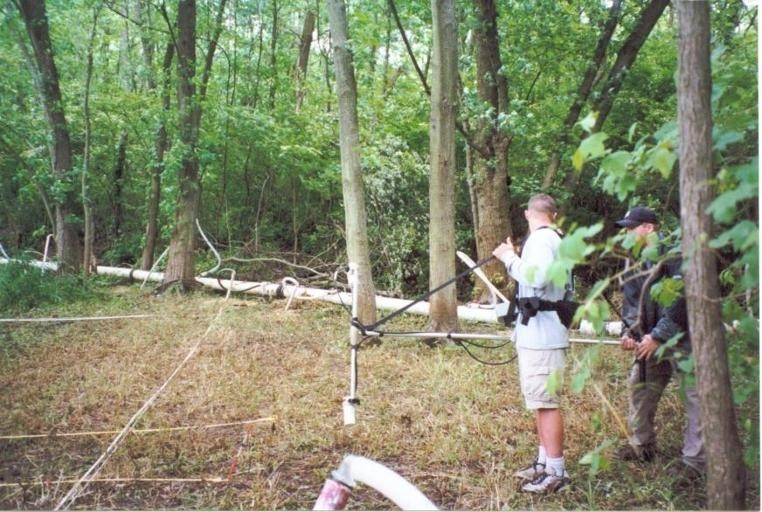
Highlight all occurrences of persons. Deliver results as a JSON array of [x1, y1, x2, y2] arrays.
[[618, 209, 705, 483], [491, 193, 573, 496]]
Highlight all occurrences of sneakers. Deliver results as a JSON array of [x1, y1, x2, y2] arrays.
[[514, 461, 572, 494]]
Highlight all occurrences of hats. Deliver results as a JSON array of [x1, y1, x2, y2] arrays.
[[616, 208, 659, 229]]
